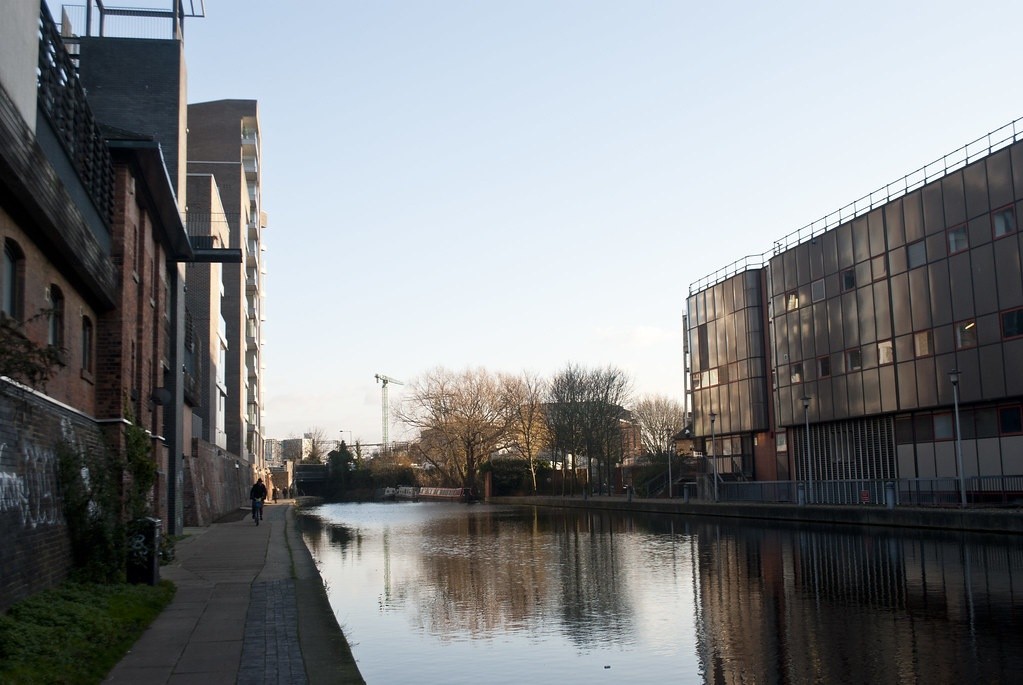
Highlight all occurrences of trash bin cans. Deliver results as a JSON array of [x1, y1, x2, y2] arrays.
[[125, 516, 164, 585]]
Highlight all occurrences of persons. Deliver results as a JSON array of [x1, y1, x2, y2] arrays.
[[250, 478, 267, 519], [283, 487, 288, 499], [273, 488, 278, 503], [289, 486, 293, 499]]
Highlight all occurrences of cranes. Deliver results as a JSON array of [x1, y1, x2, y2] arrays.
[[374, 373, 405, 455]]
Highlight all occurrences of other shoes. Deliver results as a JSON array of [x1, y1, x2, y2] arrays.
[[252, 515, 255, 519], [260, 516, 263, 520]]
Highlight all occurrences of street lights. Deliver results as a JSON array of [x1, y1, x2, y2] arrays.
[[707, 411, 719, 502], [339, 430, 352, 447], [800, 395, 814, 503], [665, 427, 672, 499], [947, 368, 968, 509]]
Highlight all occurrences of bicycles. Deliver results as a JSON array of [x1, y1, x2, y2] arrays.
[[249, 497, 265, 526]]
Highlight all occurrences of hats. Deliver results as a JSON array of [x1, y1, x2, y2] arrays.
[[257, 478, 263, 482]]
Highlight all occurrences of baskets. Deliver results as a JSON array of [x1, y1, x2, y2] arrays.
[[254, 501, 263, 508]]
